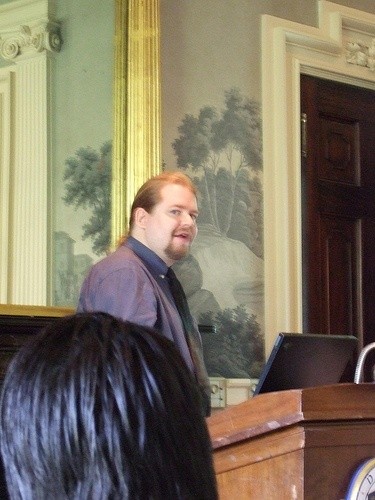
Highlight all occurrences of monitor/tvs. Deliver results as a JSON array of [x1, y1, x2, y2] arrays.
[[253, 332, 359, 397]]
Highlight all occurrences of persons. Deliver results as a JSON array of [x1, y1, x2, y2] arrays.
[[74, 171, 212, 418], [0, 311, 220, 500]]
